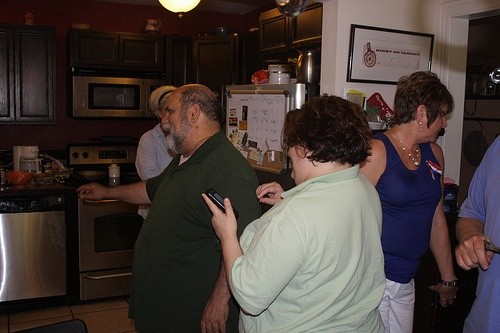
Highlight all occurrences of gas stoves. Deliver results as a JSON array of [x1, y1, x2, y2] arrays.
[[68, 141, 142, 187]]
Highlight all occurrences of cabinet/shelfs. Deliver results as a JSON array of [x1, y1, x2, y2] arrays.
[[258, 2, 321, 51], [66, 28, 162, 77], [0, 23, 57, 126], [194, 36, 240, 93], [164, 33, 193, 89]]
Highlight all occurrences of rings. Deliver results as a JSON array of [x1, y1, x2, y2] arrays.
[[453, 296, 456, 299], [441, 301, 446, 305]]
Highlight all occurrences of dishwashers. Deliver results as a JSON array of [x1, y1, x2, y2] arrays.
[[0, 196, 68, 314]]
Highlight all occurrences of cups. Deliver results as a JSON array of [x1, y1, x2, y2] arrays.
[[268, 65, 291, 84], [268, 150, 280, 168], [108, 164, 120, 178], [109, 177, 120, 187]]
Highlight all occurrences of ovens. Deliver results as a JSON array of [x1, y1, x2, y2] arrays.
[[65, 191, 152, 304]]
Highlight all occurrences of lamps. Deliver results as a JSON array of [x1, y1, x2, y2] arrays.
[[158, 0, 201, 19]]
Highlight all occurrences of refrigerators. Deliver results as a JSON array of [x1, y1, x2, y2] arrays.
[[221, 81, 319, 171]]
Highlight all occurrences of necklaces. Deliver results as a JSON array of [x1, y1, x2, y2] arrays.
[[392, 129, 419, 166]]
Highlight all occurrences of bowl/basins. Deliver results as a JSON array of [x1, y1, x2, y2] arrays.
[[76, 171, 106, 181], [366, 121, 385, 130], [33, 158, 74, 186], [5, 171, 34, 186]]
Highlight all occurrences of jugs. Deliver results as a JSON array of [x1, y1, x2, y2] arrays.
[[296, 47, 321, 85], [346, 88, 365, 110]]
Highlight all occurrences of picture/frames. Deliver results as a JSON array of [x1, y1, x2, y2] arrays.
[[346, 25, 435, 85]]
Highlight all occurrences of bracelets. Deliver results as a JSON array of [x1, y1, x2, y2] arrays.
[[441, 281, 456, 286]]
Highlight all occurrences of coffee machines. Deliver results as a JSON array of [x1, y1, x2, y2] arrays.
[[12, 144, 43, 177]]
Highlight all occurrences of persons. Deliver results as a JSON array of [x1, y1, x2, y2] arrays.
[[359, 72, 459, 333], [456, 134, 500, 333], [76, 84, 260, 333], [202, 95, 386, 333], [137, 86, 177, 234]]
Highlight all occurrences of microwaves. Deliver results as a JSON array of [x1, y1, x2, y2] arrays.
[[67, 69, 165, 119]]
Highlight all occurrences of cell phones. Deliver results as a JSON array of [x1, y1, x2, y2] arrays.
[[206, 188, 239, 217]]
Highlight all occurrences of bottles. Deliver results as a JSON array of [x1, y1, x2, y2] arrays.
[[367, 92, 396, 127]]
